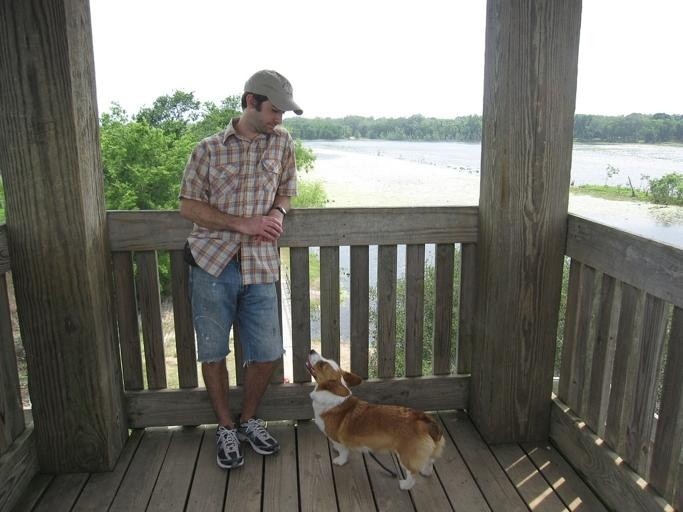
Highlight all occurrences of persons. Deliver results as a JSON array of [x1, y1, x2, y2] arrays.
[[177, 69, 306, 471]]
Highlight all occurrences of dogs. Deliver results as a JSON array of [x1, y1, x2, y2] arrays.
[[305, 349, 446, 490]]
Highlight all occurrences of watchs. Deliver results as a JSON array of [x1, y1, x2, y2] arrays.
[[271, 204, 288, 218]]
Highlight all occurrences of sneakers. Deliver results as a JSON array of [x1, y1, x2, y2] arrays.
[[216, 422, 245, 469], [237, 413, 281, 455]]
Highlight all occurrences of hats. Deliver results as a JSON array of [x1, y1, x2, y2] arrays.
[[243, 69, 303, 115]]
